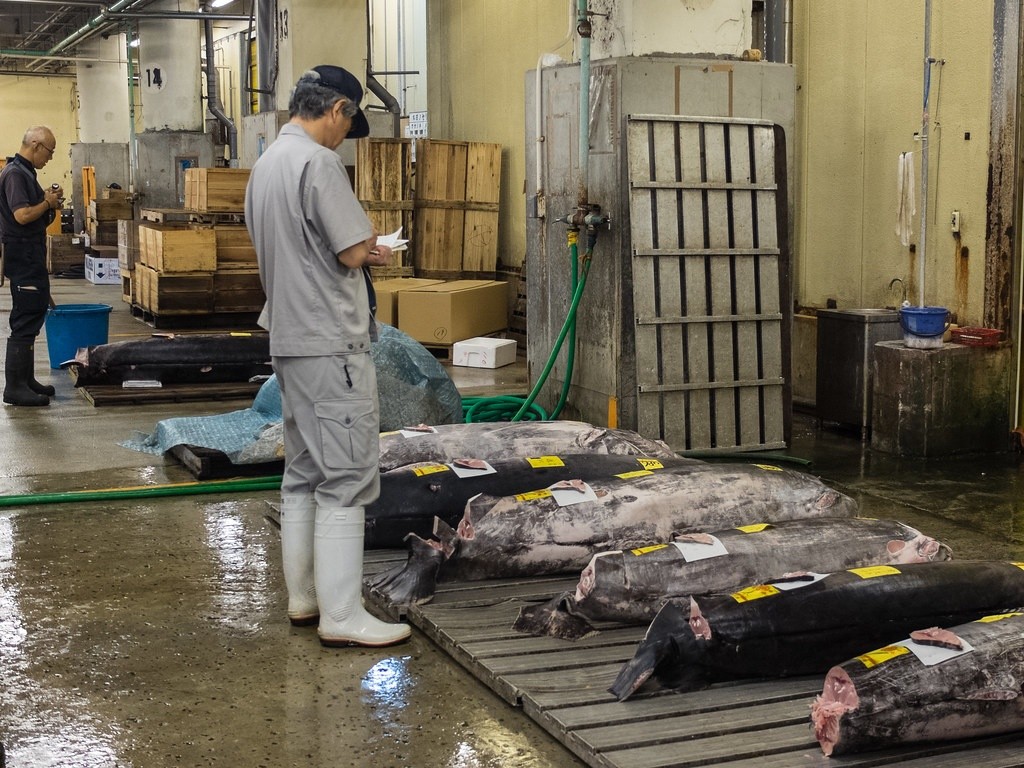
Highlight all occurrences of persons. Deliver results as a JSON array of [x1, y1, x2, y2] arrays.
[[0, 124, 64, 409], [243, 64, 414, 650]]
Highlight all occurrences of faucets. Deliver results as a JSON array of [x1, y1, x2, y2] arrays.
[[572, 203, 611, 233], [888, 277, 906, 307], [553, 213, 583, 234]]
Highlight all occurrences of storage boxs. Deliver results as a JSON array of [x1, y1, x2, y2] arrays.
[[369, 277, 446, 327], [46, 168, 268, 314], [453, 337, 517, 369], [397, 280, 509, 345]]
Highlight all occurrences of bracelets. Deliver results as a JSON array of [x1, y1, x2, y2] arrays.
[[45, 199, 52, 210]]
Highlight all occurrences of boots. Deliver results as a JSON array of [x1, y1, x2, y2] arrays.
[[281, 494, 320, 627], [313, 507, 411, 648], [2, 337, 56, 407]]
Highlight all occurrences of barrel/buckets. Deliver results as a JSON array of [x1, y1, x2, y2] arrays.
[[45, 304, 113, 369], [897, 306, 951, 348]]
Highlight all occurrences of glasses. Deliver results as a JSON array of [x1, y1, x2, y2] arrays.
[[32, 141, 54, 154]]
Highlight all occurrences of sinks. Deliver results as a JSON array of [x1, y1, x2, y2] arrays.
[[816, 309, 900, 315]]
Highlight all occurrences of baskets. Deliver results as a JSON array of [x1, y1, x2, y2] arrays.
[[949, 326, 1005, 348]]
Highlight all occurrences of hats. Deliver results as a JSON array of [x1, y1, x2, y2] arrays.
[[298, 65, 370, 139]]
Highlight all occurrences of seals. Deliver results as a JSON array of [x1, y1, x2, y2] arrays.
[[59, 331, 272, 390], [363, 421, 1024, 758]]
